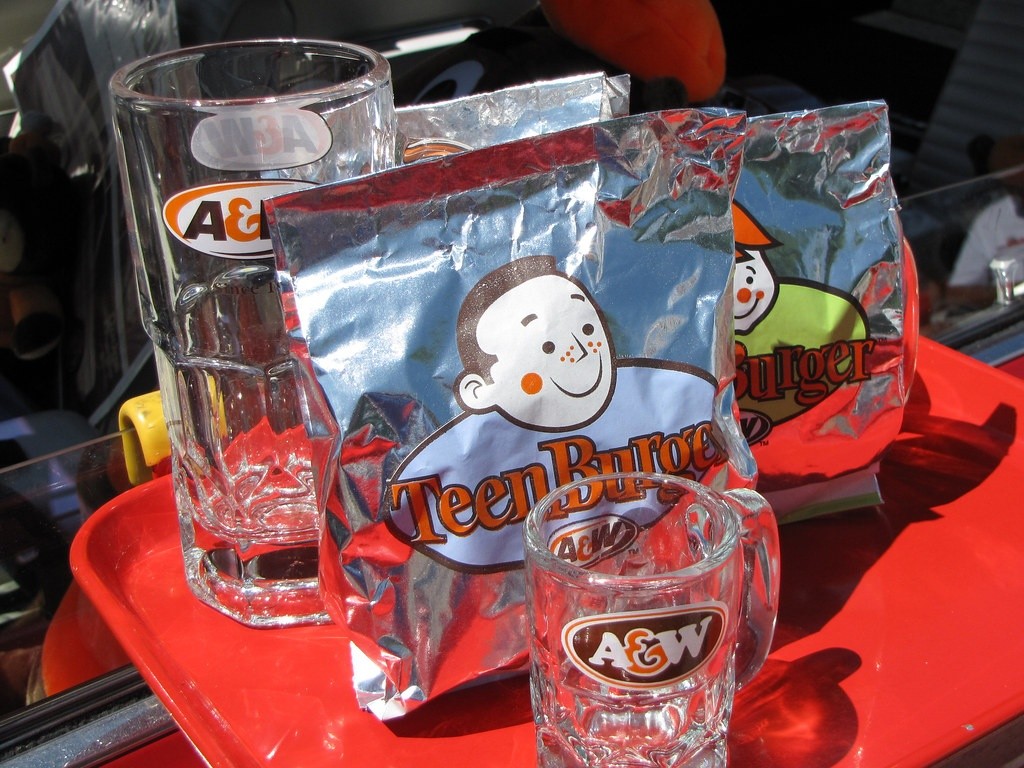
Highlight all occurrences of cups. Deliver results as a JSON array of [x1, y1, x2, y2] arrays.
[[523, 470, 783, 767], [109, 39, 394, 629]]
